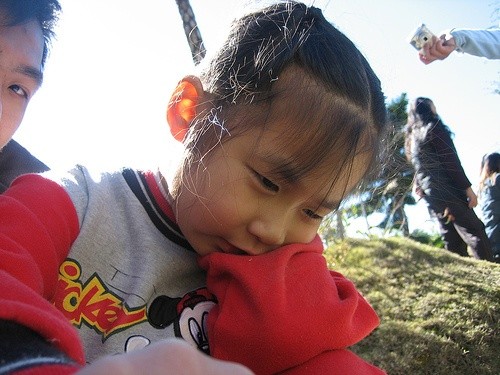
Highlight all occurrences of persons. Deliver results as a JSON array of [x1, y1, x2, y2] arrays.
[[404, 98, 494, 263], [0, 1, 390, 375], [418, 28, 500, 65], [478, 153, 500, 264], [1, 1, 62, 194]]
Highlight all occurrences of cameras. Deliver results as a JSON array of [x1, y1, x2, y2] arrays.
[[409, 23, 440, 57]]
